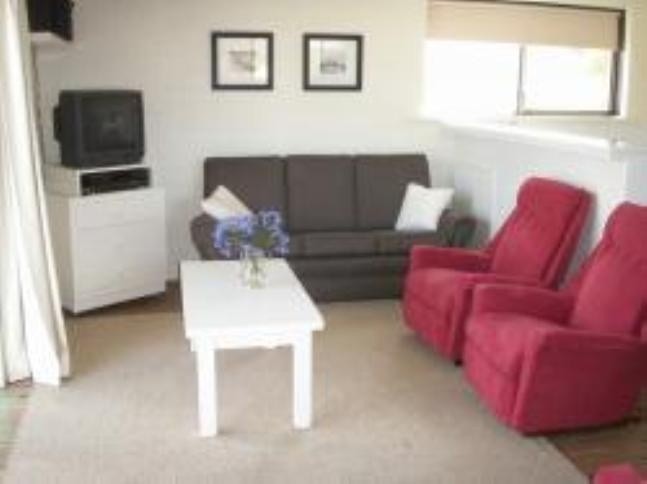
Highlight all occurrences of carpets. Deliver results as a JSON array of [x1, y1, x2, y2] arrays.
[[2, 298, 591, 484]]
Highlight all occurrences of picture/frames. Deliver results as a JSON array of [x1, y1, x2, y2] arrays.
[[211, 31, 362, 89]]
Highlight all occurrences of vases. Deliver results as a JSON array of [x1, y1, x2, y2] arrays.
[[238, 245, 266, 288]]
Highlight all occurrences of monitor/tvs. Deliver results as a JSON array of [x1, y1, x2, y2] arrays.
[[53, 90, 144, 167]]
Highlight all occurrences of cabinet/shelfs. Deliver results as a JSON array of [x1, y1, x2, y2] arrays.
[[46, 161, 166, 317]]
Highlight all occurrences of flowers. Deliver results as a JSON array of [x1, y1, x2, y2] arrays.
[[211, 210, 291, 281]]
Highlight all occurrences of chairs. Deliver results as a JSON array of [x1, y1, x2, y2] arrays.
[[459, 197, 646, 435], [405, 179, 595, 366]]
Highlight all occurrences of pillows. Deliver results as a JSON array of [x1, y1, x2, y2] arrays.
[[199, 184, 255, 223], [395, 181, 455, 232]]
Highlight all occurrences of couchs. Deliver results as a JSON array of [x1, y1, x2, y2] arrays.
[[191, 155, 478, 300]]
